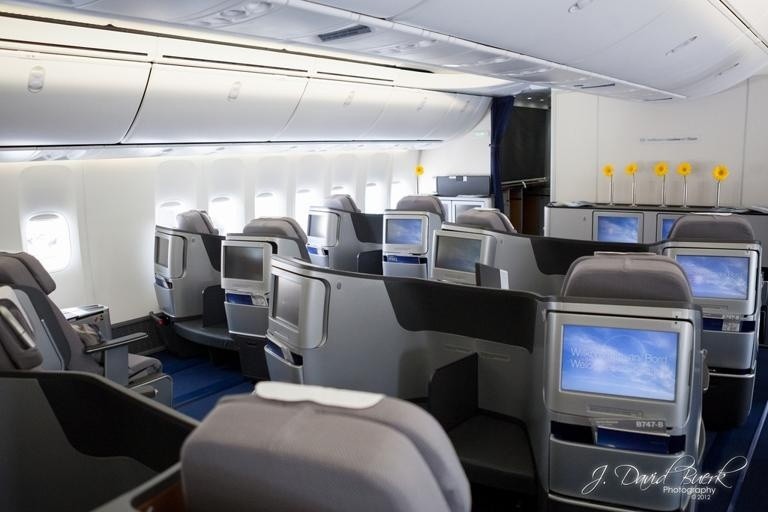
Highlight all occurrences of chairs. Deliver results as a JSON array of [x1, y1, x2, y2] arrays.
[[1, 194, 767, 511]]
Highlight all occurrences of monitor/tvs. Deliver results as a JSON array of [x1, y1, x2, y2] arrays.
[[451, 201, 485, 224], [268, 266, 326, 349], [657, 214, 682, 242], [593, 211, 644, 243], [661, 247, 759, 315], [439, 201, 451, 222], [546, 311, 694, 427], [306, 211, 337, 246], [382, 215, 429, 253], [153, 231, 184, 278], [431, 228, 496, 284], [220, 239, 273, 294]]
